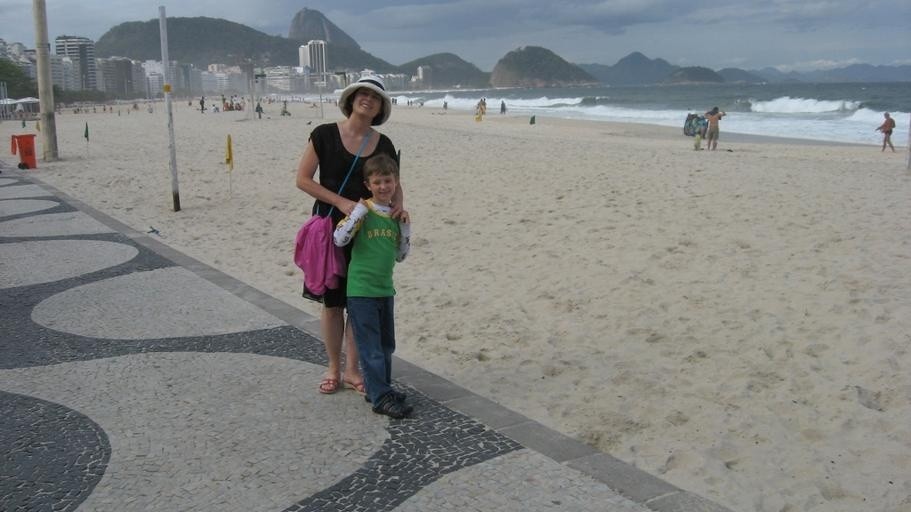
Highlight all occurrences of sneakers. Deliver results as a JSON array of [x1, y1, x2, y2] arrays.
[[365, 389, 407, 403], [373, 396, 412, 419]]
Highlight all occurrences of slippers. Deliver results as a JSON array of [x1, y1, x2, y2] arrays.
[[318, 373, 343, 394], [340, 377, 367, 396]]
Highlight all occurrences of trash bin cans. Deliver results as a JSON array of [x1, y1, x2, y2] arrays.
[[11, 134, 36, 169]]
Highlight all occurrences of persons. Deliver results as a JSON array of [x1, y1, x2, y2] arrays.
[[333, 152, 415, 419], [692, 125, 703, 149], [294, 74, 399, 393], [874, 111, 899, 154], [704, 105, 727, 150], [500, 100, 507, 114]]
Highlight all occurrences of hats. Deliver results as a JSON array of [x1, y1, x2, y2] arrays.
[[337, 74, 392, 126]]
